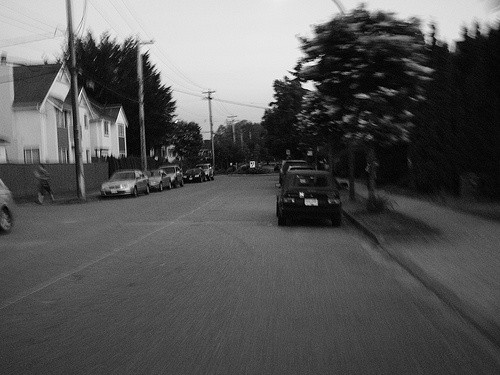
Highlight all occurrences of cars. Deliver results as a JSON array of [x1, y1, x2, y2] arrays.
[[145, 169, 172, 191], [183, 168, 205, 183], [100, 169, 150, 197], [277, 170, 341, 226], [286, 165, 314, 172], [159, 166, 184, 187], [196, 163, 215, 180], [236, 164, 264, 174], [1, 178, 16, 232], [279, 159, 307, 184]]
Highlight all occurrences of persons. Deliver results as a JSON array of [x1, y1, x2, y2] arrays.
[[33, 164, 57, 205]]
